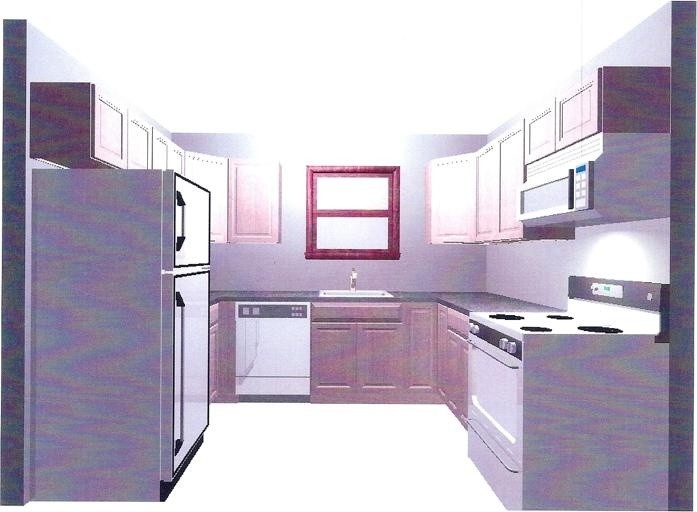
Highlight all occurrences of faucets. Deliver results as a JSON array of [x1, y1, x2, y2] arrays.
[[349, 267, 358, 292]]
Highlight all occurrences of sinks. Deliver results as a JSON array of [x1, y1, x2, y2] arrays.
[[318, 288, 395, 299]]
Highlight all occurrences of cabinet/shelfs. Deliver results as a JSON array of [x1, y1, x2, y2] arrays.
[[179, 147, 229, 247], [436, 302, 471, 429], [424, 153, 478, 248], [225, 155, 283, 249], [208, 299, 219, 406], [474, 118, 576, 244], [145, 119, 184, 178], [309, 301, 435, 403], [28, 78, 150, 179], [527, 63, 670, 168]]
